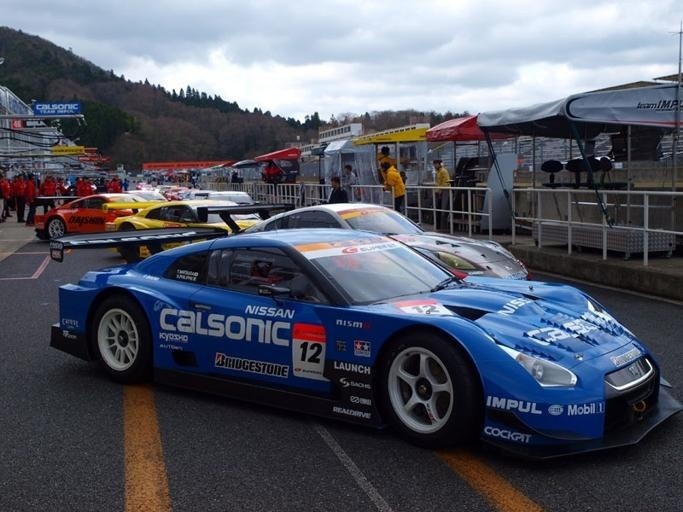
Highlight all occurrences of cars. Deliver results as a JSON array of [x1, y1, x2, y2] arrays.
[[142, 168, 261, 181]]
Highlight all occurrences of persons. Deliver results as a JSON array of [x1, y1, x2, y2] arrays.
[[380, 162, 405, 211], [376, 145, 396, 183], [0, 170, 130, 225], [399, 170, 407, 185], [230, 172, 239, 185], [328, 177, 347, 203], [144, 168, 200, 188], [433, 158, 451, 230], [341, 165, 356, 197]]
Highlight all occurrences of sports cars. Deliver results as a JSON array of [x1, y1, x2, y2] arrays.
[[44, 227, 679, 461]]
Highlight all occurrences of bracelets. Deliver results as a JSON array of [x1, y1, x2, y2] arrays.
[[382, 187, 385, 191]]
